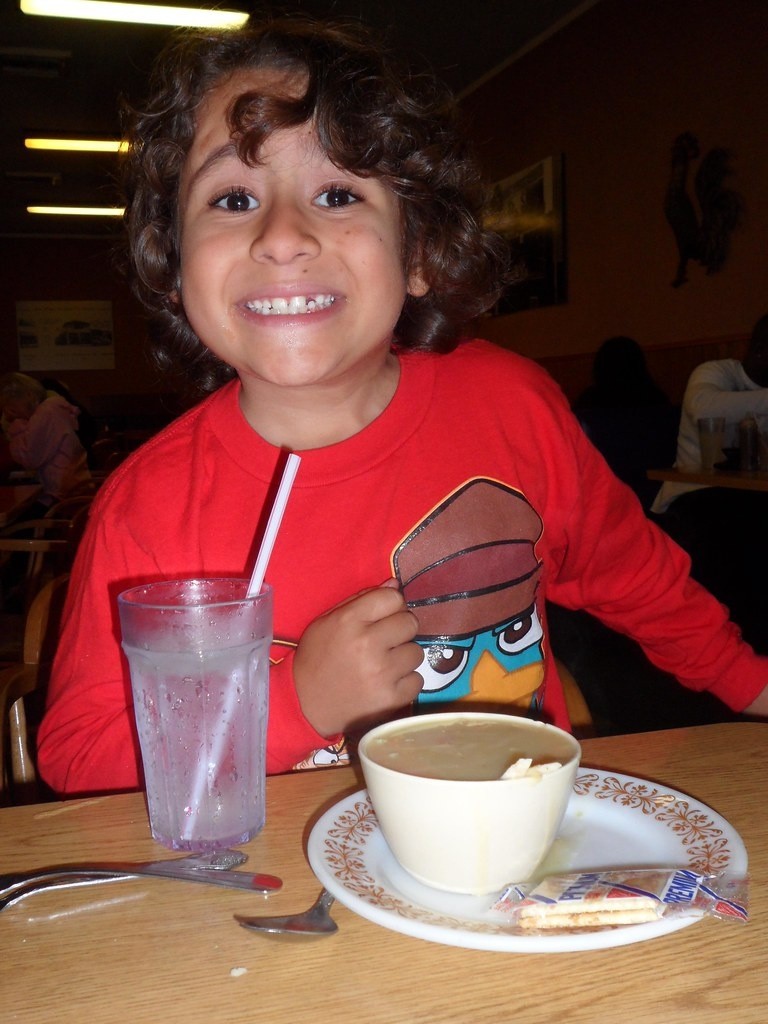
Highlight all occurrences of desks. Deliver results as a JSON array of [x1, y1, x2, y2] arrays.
[[645, 469, 768, 495]]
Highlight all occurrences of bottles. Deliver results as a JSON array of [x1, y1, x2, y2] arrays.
[[736, 413, 758, 473]]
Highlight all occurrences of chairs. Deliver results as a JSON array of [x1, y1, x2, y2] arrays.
[[0, 490, 98, 810]]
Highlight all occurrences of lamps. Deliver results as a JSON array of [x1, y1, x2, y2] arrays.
[[26, 128, 129, 157], [19, 0, 251, 37], [23, 196, 128, 219]]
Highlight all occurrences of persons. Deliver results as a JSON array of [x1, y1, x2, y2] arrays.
[[571, 313, 768, 515], [0, 370, 93, 519], [35, 23, 768, 799]]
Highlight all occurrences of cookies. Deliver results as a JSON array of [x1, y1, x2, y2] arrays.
[[519, 871, 674, 928]]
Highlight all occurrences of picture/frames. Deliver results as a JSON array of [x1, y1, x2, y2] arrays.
[[483, 152, 571, 320]]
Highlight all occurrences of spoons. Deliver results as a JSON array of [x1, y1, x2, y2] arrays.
[[237, 887, 340, 943]]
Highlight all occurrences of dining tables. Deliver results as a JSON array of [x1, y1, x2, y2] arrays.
[[0, 722, 768, 1024]]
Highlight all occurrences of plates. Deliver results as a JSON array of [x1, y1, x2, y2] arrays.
[[306, 768, 748, 954]]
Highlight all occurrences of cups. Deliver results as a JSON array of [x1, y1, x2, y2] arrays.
[[698, 417, 726, 469], [118, 577, 273, 852], [357, 712, 582, 894]]
